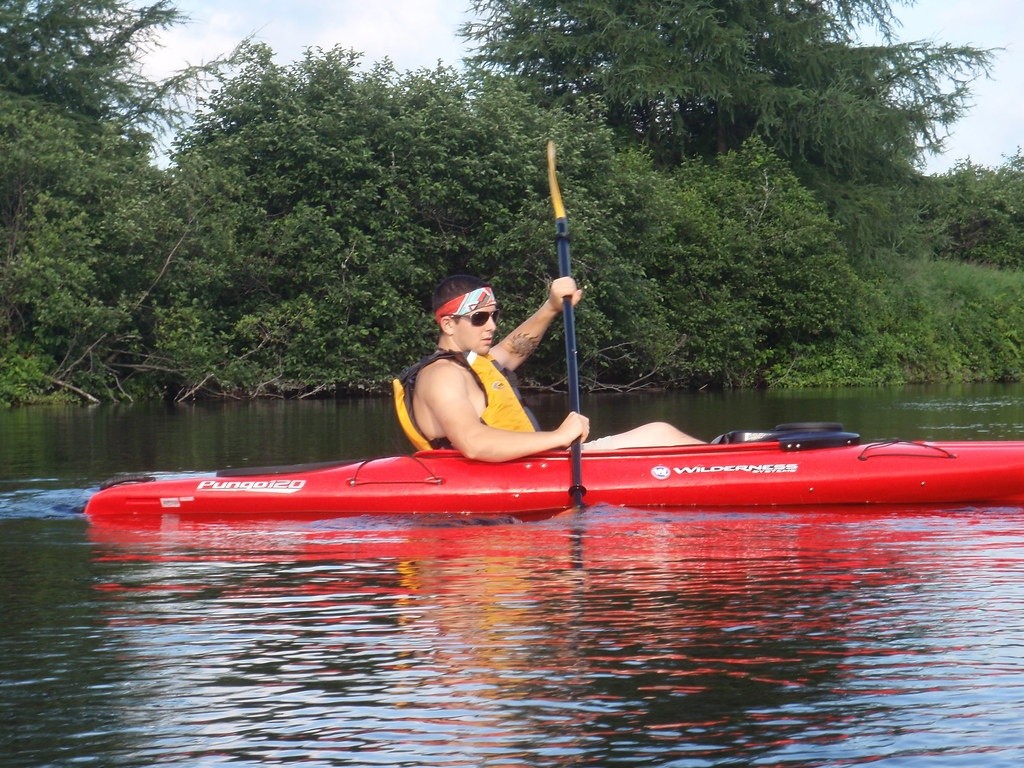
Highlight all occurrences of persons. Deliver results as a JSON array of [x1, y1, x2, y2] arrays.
[[412, 274, 711, 463]]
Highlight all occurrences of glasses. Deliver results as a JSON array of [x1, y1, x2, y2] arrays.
[[442, 309, 500, 326]]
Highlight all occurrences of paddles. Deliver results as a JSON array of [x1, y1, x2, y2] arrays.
[[543, 135, 589, 516]]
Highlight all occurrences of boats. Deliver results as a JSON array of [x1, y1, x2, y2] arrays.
[[81, 423, 1024, 523]]
